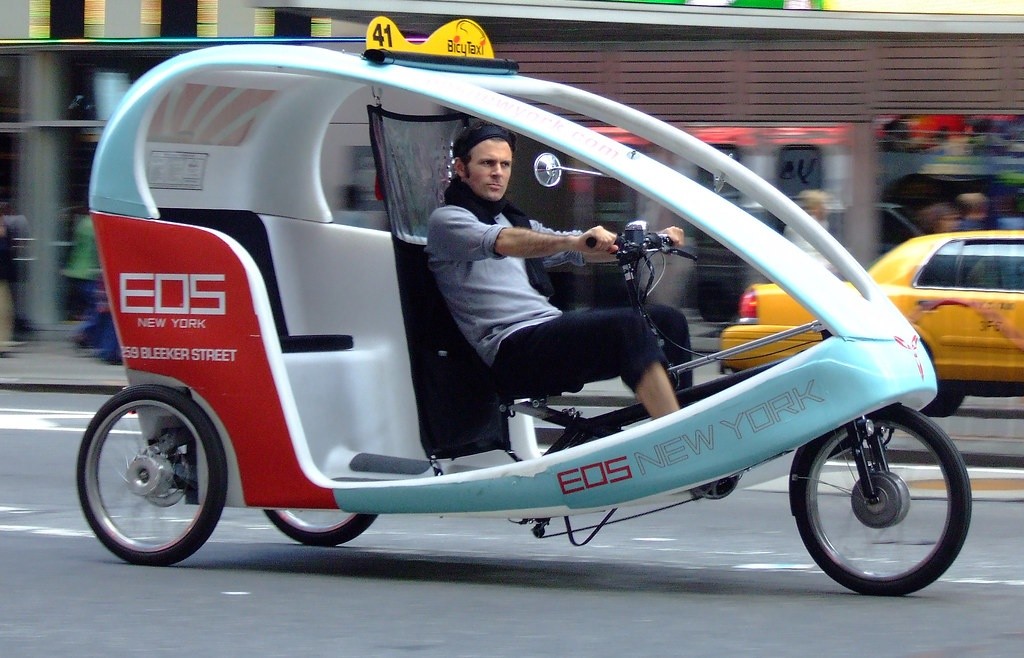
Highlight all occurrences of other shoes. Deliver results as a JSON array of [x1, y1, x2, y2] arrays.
[[690, 471, 740, 499]]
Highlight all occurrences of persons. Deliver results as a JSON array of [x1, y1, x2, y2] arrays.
[[776, 189, 1024, 291], [0, 192, 124, 366], [425, 120, 693, 422]]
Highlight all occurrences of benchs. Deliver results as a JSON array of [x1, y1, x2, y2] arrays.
[[94, 195, 354, 352]]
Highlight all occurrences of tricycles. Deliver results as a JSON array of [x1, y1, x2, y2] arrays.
[[74, 15, 973, 597]]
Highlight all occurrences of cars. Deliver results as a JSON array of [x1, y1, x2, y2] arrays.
[[718, 230, 1024, 419]]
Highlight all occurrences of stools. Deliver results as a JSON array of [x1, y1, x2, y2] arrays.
[[436, 346, 585, 453]]
